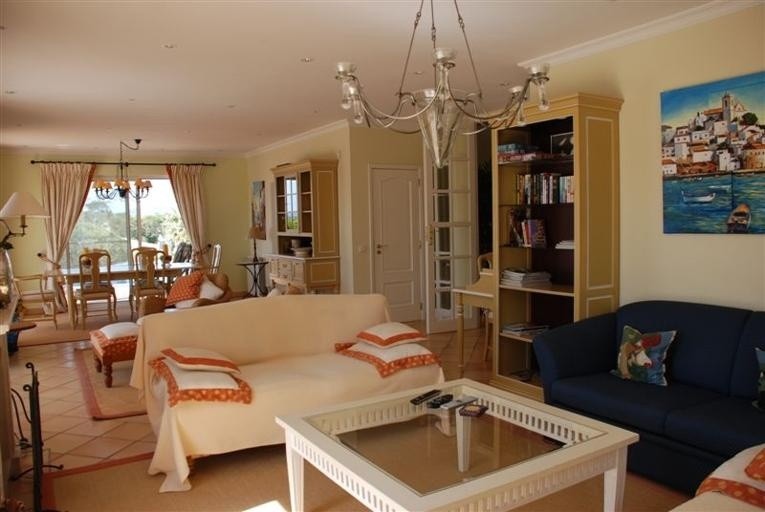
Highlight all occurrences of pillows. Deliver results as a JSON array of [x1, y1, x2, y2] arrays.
[[749, 340, 765, 413], [611, 325, 679, 391], [746, 443, 765, 482], [357, 321, 431, 348], [158, 342, 245, 375], [147, 363, 254, 409], [335, 339, 446, 379], [695, 443, 765, 509]]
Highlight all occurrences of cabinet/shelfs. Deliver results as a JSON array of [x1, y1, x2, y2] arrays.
[[267, 158, 340, 294], [483, 92, 627, 401]]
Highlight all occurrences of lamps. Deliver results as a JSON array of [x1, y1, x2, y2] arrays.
[[92, 140, 151, 200], [334, 0, 555, 172], [1, 192, 51, 251]]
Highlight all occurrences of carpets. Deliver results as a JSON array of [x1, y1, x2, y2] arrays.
[[44, 421, 690, 512]]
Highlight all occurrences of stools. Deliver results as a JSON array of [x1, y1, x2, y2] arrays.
[[87, 321, 137, 390]]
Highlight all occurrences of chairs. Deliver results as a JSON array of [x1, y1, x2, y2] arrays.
[[15, 242, 225, 330]]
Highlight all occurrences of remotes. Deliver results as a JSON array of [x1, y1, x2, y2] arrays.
[[440, 396, 478, 410], [426, 393, 453, 408], [410, 389, 442, 405]]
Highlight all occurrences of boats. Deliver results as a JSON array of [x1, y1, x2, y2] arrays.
[[681, 190, 717, 206], [706, 184, 732, 193], [727, 202, 752, 234]]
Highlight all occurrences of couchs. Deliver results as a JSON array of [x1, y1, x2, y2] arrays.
[[137, 293, 447, 494], [665, 492, 762, 512], [528, 298, 764, 496]]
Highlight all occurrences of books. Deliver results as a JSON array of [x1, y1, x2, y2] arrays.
[[499, 143, 574, 337]]
